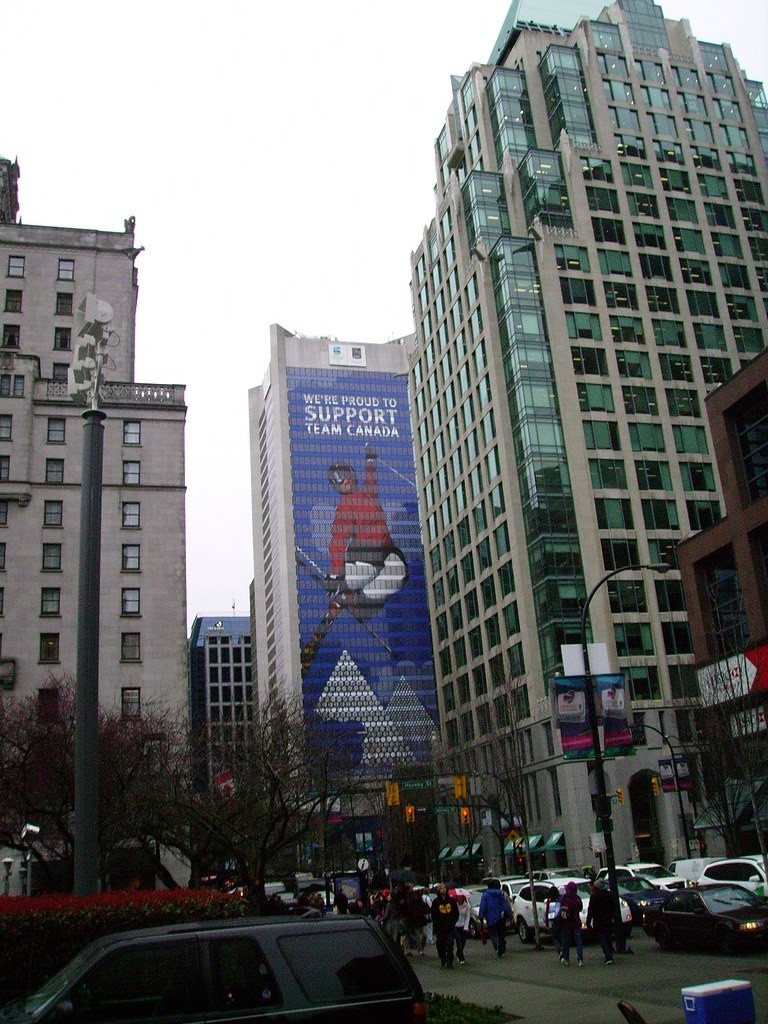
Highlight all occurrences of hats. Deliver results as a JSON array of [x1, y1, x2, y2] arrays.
[[594, 878, 604, 889]]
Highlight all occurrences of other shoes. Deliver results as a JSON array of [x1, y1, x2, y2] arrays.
[[557, 952, 564, 961], [404, 943, 508, 969], [564, 960, 569, 965], [577, 960, 584, 966], [604, 960, 612, 966]]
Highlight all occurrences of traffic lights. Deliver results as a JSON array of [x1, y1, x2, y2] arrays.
[[461, 808, 470, 825], [518, 843, 522, 851], [518, 857, 523, 866], [405, 806, 415, 822]]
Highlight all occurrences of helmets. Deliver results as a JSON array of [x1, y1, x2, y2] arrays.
[[330, 462, 358, 494]]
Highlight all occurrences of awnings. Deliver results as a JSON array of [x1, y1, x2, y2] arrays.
[[691, 773, 768, 829], [498, 829, 565, 856], [430, 842, 482, 863]]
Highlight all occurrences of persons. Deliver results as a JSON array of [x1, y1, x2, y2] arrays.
[[299, 443, 409, 682], [545, 882, 583, 967], [586, 879, 614, 966], [298, 885, 431, 954], [479, 879, 513, 958], [453, 888, 470, 964], [431, 883, 459, 970]]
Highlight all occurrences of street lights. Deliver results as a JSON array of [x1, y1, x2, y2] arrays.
[[579, 562, 671, 957], [623, 724, 692, 859], [665, 734, 708, 857], [319, 730, 370, 909]]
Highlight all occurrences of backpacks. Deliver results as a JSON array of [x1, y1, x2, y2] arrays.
[[547, 900, 568, 930]]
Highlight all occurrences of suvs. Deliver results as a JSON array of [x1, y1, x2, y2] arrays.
[[0, 909, 428, 1024]]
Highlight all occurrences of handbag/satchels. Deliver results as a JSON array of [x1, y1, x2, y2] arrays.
[[480, 919, 487, 946]]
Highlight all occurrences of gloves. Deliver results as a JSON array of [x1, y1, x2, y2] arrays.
[[323, 571, 350, 604], [364, 442, 378, 467]]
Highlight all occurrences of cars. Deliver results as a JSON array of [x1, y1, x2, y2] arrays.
[[233, 855, 768, 950]]
